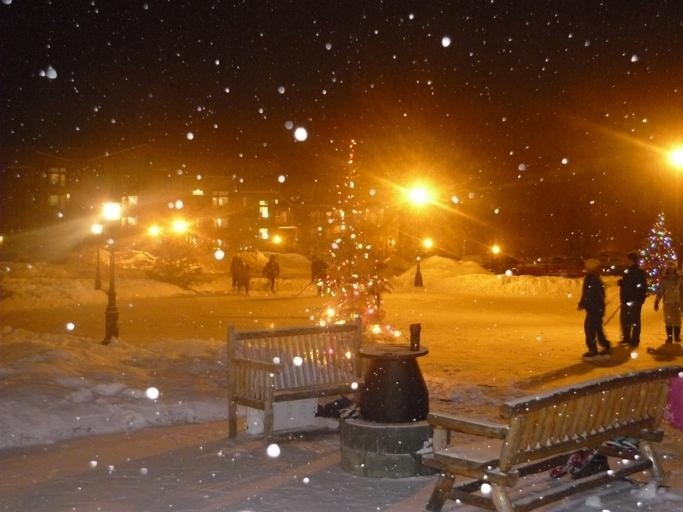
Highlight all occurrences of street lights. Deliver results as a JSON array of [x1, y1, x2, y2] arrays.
[[91, 203, 121, 345], [407, 180, 430, 287], [670, 141, 683, 246]]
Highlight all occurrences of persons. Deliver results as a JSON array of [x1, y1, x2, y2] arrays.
[[261, 252, 281, 292], [227, 252, 251, 295], [615, 250, 647, 348], [577, 258, 612, 358], [653, 260, 683, 343], [309, 255, 329, 298]]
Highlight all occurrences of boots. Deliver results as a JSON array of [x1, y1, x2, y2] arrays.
[[664, 326, 682, 343]]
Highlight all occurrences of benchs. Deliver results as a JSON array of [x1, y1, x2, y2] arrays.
[[420, 364, 683, 512], [225, 316, 365, 445]]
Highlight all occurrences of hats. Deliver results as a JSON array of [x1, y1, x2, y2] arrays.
[[583, 257, 601, 271], [625, 250, 639, 264]]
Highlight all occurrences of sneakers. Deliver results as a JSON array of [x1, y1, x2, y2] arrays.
[[584, 339, 611, 357], [618, 339, 639, 349]]
